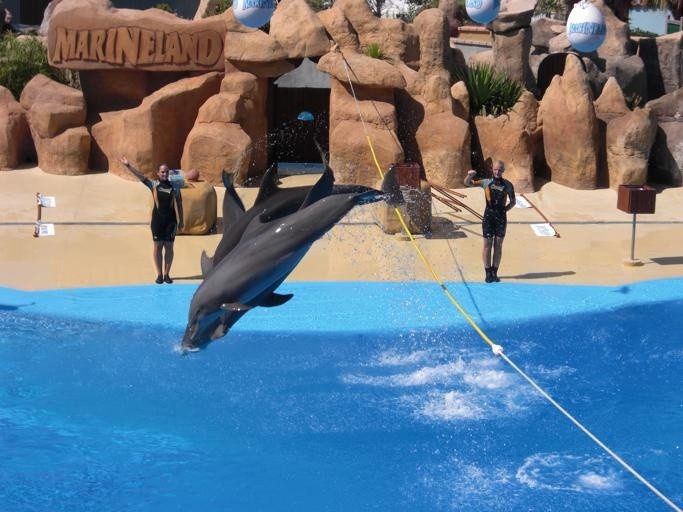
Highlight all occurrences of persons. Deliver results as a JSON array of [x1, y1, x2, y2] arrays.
[[119, 156, 185, 285], [464, 160, 517, 284]]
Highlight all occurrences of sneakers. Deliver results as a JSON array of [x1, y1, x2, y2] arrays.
[[155, 275, 164, 284], [164, 276, 174, 285]]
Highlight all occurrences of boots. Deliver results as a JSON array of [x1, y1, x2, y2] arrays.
[[491, 266, 500, 282], [483, 267, 493, 284]]
